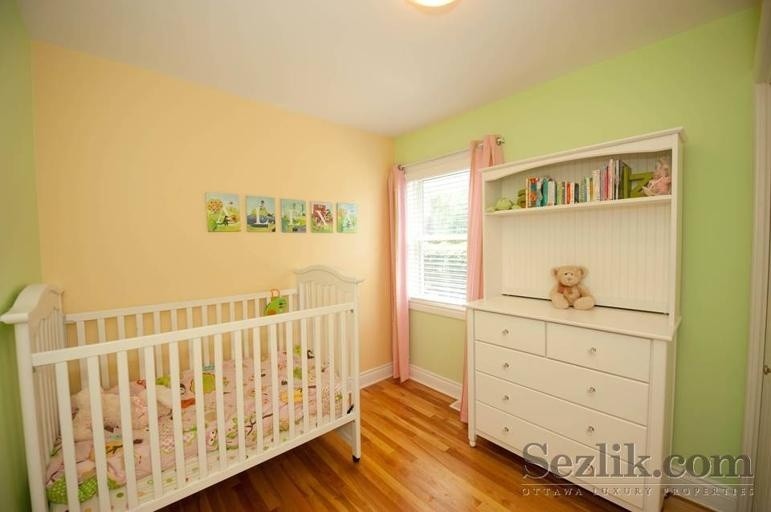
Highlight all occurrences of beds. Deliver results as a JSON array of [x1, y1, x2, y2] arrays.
[[0, 264, 367, 512]]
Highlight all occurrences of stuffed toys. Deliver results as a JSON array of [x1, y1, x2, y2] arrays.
[[487, 197, 522, 211], [548, 265, 596, 310]]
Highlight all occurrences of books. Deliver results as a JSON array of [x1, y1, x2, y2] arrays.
[[525, 157, 631, 208]]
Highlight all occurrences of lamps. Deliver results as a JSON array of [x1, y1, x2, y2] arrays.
[[407, 0, 459, 9]]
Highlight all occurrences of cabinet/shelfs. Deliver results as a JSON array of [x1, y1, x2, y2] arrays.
[[462, 127, 686, 512]]
